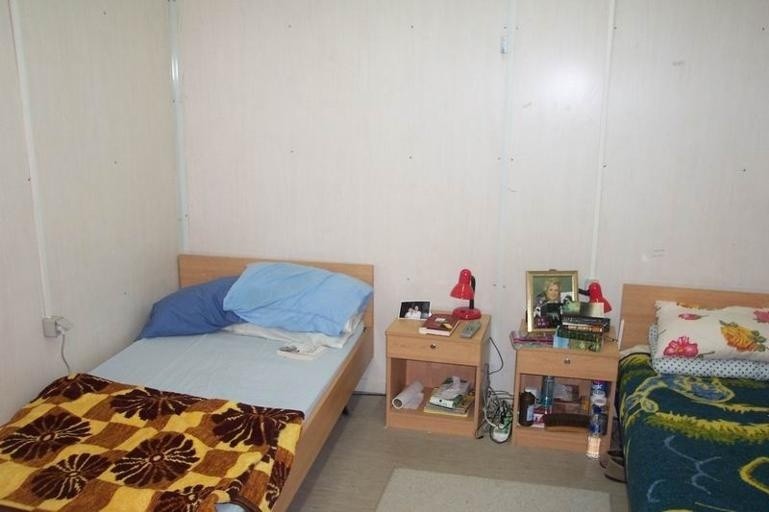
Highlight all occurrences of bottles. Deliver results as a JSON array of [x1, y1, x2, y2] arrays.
[[585, 379, 608, 459], [518, 375, 554, 427]]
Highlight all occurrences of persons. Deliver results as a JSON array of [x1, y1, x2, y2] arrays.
[[410, 305, 423, 320], [533, 276, 566, 316], [403, 306, 415, 320]]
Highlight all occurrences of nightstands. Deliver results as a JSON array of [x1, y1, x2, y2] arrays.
[[385, 310, 619, 456]]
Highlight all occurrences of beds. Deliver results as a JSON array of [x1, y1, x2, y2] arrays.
[[0, 254, 374, 510], [605, 283, 769, 512]]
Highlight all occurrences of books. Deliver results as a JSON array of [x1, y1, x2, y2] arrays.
[[423, 376, 475, 418], [418, 311, 462, 336], [551, 313, 612, 352]]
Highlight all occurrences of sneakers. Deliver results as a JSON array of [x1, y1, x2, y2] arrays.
[[605, 457, 625, 483], [599, 447, 624, 468]]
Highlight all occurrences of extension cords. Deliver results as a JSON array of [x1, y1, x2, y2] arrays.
[[492, 402, 513, 441]]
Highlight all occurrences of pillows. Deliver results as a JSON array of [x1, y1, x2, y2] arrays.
[[132, 261, 373, 348], [646, 298, 769, 383]]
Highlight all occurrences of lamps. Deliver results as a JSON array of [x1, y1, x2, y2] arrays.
[[573, 276, 615, 317], [450, 268, 482, 321]]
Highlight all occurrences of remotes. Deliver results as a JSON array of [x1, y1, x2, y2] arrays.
[[461, 319, 481, 338]]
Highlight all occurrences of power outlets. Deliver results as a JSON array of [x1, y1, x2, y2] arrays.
[[42, 314, 76, 339]]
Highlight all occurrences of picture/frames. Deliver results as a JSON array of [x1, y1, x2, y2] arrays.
[[525, 269, 579, 334]]
